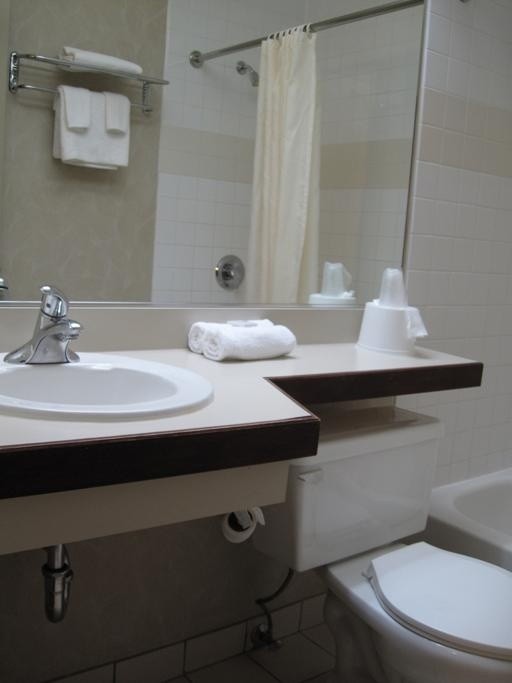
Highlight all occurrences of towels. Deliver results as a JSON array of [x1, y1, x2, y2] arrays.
[[51, 44, 141, 171], [187, 315, 297, 363]]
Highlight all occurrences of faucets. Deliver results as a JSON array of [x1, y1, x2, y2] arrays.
[[0, 285, 84, 364]]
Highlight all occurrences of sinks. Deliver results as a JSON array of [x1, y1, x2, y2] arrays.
[[1, 353, 215, 415]]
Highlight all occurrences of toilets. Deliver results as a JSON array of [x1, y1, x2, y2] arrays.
[[258, 406, 512, 683]]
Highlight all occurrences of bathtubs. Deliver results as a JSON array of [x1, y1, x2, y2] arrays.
[[426, 466, 512, 570]]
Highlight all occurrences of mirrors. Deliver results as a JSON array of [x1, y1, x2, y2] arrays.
[[0, 0, 428, 306]]
